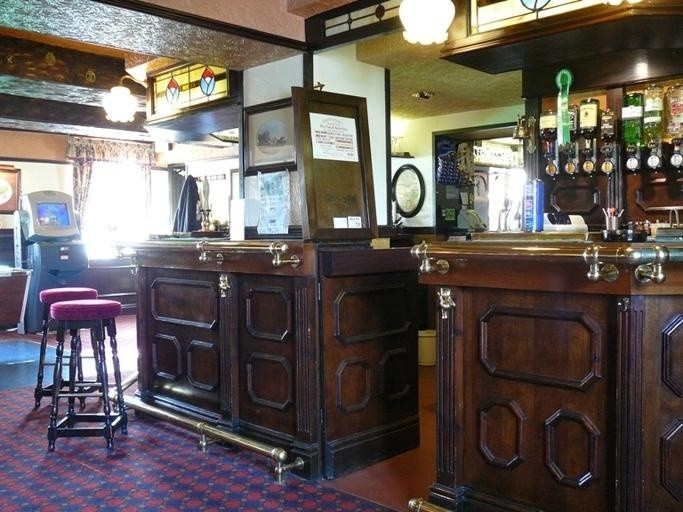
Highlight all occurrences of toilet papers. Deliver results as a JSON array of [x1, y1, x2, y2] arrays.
[[228, 199, 246, 241]]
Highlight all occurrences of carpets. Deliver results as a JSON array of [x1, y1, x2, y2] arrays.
[[2, 377, 405, 510]]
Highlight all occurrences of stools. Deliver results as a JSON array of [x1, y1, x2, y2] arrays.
[[34, 285, 133, 450]]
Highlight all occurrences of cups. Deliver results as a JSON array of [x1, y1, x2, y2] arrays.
[[605, 216, 618, 231]]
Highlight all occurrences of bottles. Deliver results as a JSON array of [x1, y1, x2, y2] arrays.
[[540, 98, 616, 179], [623, 85, 683, 170], [628, 220, 649, 234]]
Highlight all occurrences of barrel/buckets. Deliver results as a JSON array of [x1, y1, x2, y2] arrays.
[[417, 330, 437, 366]]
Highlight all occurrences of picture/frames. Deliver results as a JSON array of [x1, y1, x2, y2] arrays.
[[0, 166, 23, 214], [239, 101, 297, 177]]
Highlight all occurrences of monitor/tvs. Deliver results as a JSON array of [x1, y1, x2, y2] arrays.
[[37, 203, 69, 226]]
